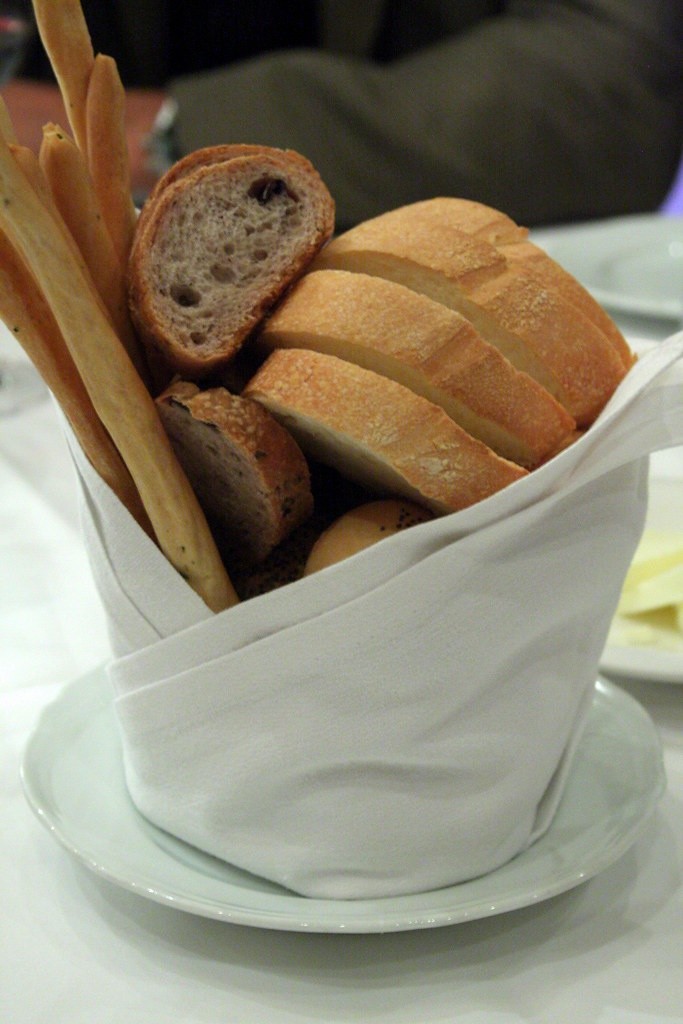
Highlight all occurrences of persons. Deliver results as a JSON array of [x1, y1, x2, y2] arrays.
[[0, 0, 683, 236]]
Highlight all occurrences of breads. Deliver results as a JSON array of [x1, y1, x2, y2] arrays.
[[0, 0, 634, 612]]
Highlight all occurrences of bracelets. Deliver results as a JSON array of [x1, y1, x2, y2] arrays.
[[150, 95, 181, 171]]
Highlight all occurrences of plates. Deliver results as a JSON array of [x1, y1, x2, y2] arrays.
[[595, 475, 683, 682], [19, 659, 662, 932]]
